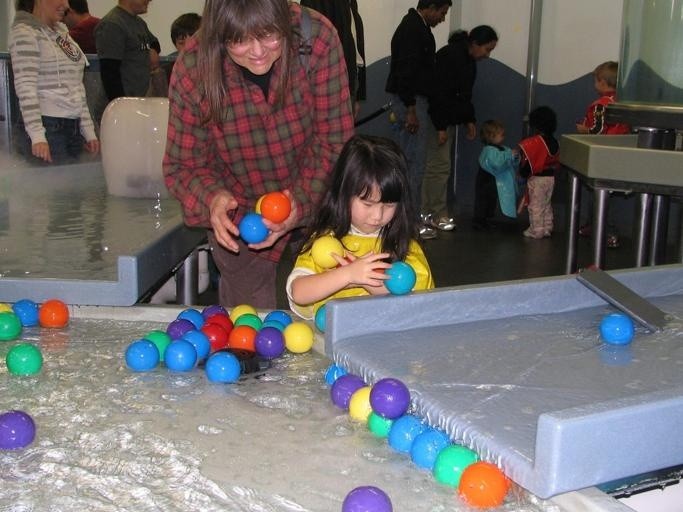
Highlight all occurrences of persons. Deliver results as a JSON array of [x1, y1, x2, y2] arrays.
[[284, 132, 435, 319], [574, 60, 634, 134], [12, 0, 106, 169], [473, 118, 519, 232], [94, 0, 162, 102], [517, 104, 559, 241], [381, 0, 454, 180], [59, 0, 105, 54], [420, 23, 500, 238], [148, 11, 204, 99], [162, 0, 353, 311]]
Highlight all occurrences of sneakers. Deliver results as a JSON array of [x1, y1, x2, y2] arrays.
[[419, 225, 435, 239], [421, 213, 430, 224], [544, 230, 551, 237], [524, 230, 543, 239], [430, 217, 456, 231]]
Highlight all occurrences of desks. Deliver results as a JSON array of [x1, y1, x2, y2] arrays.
[[1, 162, 220, 306], [1, 299, 638, 511]]
